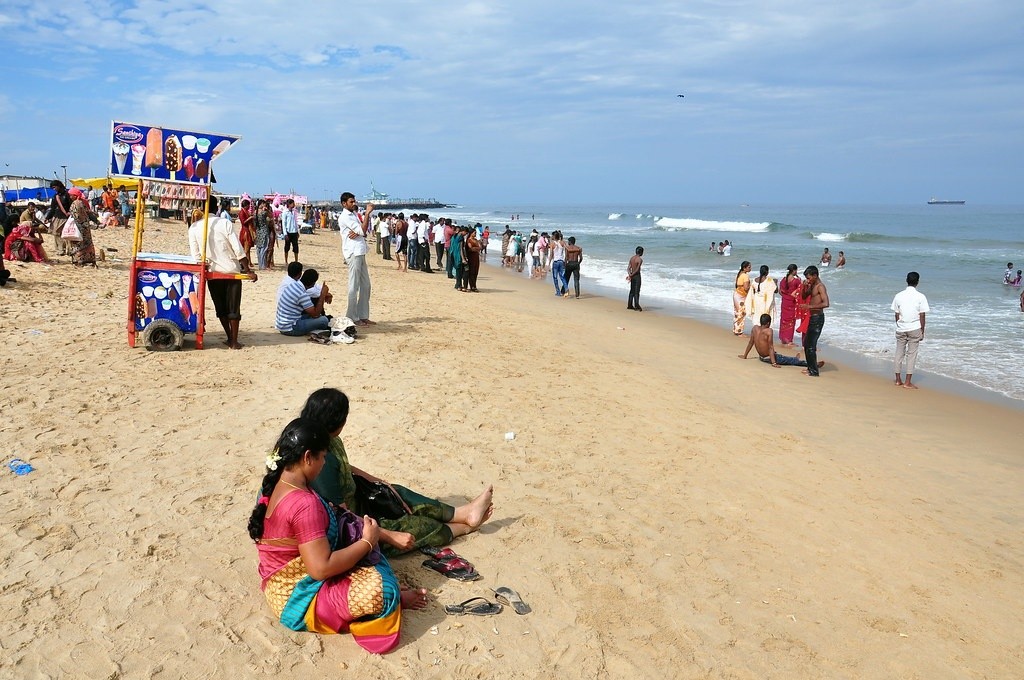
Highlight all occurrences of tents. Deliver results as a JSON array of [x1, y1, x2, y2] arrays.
[[68, 177, 144, 195]]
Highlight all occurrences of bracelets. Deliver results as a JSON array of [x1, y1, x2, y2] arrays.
[[360, 539, 373, 554]]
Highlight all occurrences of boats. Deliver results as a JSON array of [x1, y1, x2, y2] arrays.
[[927, 195, 966, 205]]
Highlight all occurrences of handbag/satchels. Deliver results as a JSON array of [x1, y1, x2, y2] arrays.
[[353, 475, 405, 520], [61, 215, 83, 242], [333, 506, 381, 566]]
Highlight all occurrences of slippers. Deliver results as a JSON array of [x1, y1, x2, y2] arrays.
[[491, 586, 526, 615], [444, 596, 502, 616], [423, 558, 480, 582], [421, 546, 468, 564]]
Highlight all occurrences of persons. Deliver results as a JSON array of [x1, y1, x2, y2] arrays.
[[1003, 262, 1013, 285], [189, 194, 257, 349], [1020, 290, 1024, 312], [252, 389, 497, 556], [733, 261, 829, 378], [1012, 270, 1022, 286], [888, 271, 929, 389], [818, 248, 832, 267], [834, 251, 845, 268], [254, 414, 431, 654], [625, 246, 644, 312], [0, 180, 585, 337], [709, 239, 732, 257]]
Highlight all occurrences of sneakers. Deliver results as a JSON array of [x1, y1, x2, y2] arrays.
[[329, 330, 355, 343]]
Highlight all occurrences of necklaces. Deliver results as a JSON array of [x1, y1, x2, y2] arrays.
[[279, 477, 307, 492]]
